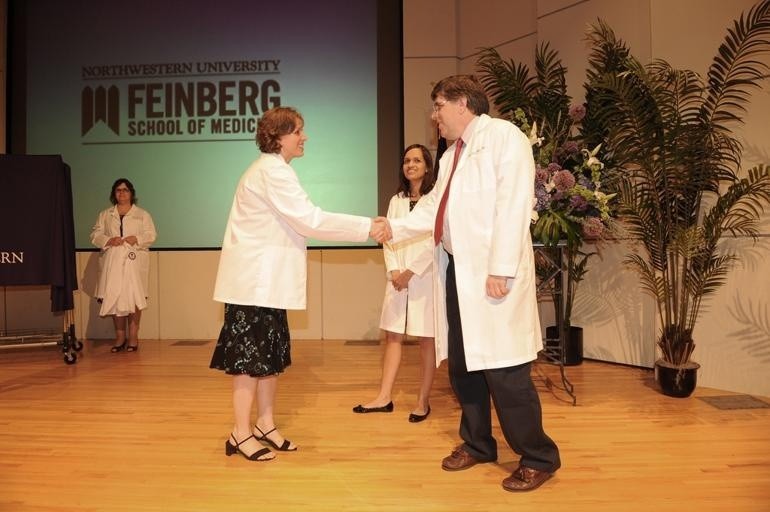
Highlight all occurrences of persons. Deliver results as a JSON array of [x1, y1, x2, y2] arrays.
[[88, 177, 156, 354], [353, 144, 436, 423], [367, 74, 562, 493], [207, 106, 393, 462]]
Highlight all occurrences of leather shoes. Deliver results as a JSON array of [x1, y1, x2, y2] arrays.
[[408, 406, 431, 423], [501, 464, 555, 492], [441, 448, 480, 471], [353, 401, 393, 413]]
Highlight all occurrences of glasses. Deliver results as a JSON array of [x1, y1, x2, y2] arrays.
[[432, 98, 448, 112]]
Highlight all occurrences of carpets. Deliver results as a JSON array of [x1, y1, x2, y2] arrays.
[[698, 394, 770, 411]]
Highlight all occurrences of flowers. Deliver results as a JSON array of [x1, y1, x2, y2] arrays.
[[508, 107, 640, 257]]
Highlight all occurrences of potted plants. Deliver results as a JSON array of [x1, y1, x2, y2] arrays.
[[470, 40, 619, 366], [574, 1, 770, 398]]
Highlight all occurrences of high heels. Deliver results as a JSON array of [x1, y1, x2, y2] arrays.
[[226, 432, 276, 462], [111, 338, 127, 353], [252, 425, 298, 452], [127, 339, 138, 353]]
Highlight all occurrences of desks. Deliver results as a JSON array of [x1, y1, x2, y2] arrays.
[[528, 240, 578, 405]]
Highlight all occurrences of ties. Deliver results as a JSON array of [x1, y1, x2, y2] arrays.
[[433, 138, 464, 246]]
